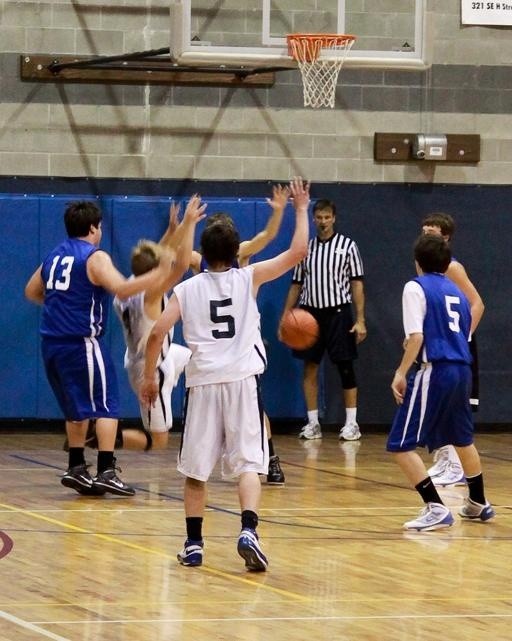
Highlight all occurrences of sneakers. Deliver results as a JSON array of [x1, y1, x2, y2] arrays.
[[175, 540, 205, 567], [400, 502, 455, 535], [456, 497, 496, 522], [91, 467, 137, 497], [236, 528, 270, 572], [338, 421, 362, 442], [298, 420, 323, 440], [63, 419, 96, 452], [61, 463, 107, 496], [427, 453, 449, 477], [424, 446, 468, 489], [265, 454, 285, 486]]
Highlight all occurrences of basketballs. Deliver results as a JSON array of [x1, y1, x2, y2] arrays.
[[280, 308, 317, 349]]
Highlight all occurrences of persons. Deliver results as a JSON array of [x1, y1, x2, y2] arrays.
[[25, 200, 173, 495], [386, 233, 495, 532], [403, 212, 484, 488], [158, 183, 293, 486], [277, 199, 367, 442], [62, 195, 207, 452], [138, 175, 311, 573]]
